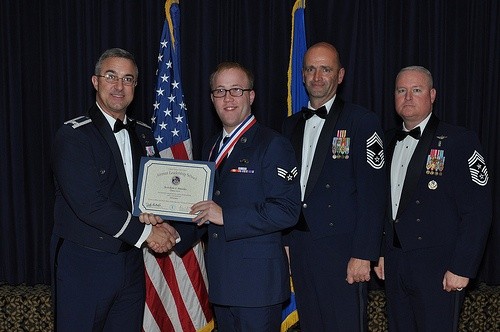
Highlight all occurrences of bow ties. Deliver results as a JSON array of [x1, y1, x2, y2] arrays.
[[113, 118, 137, 133], [302, 105, 327, 120], [395, 126, 421, 142]]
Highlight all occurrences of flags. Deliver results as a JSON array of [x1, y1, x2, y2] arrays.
[[142, 0, 216, 332], [280, 0, 314, 332], [51, 48, 175, 332]]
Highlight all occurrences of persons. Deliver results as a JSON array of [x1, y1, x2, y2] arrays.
[[373, 65, 491, 332], [147, 63, 302, 332], [280, 41, 387, 332]]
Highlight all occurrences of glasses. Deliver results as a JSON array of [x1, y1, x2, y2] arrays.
[[211, 88, 252, 98], [95, 72, 137, 86]]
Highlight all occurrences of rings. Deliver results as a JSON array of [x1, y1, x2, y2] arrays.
[[457, 288, 463, 291]]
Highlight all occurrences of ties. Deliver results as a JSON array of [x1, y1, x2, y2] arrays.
[[222, 137, 230, 159]]
